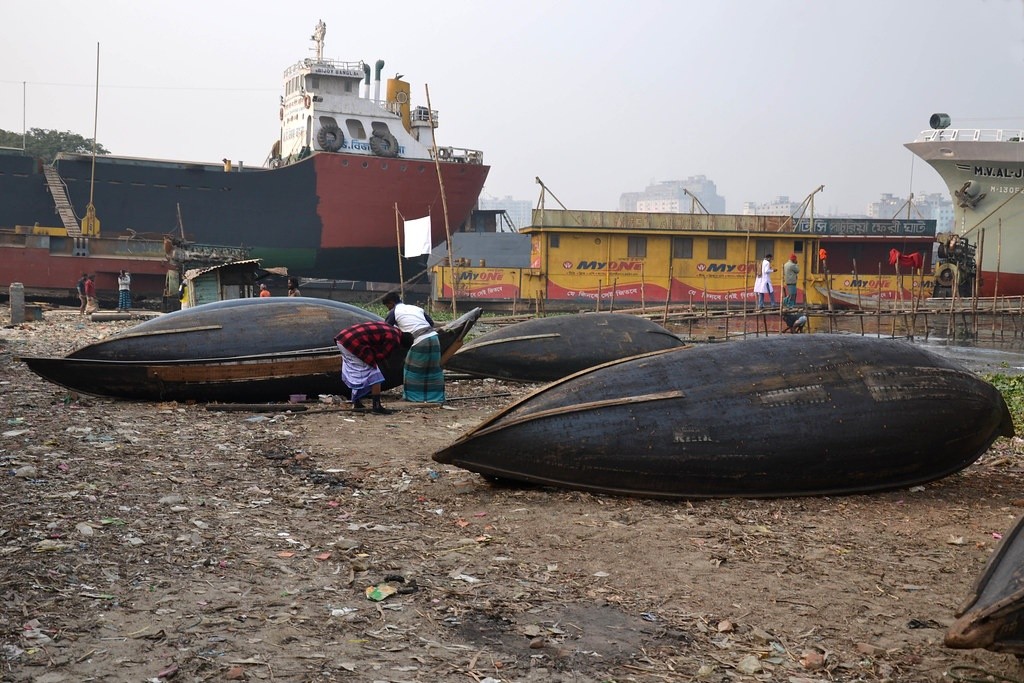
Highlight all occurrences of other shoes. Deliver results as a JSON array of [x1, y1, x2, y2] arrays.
[[352, 405, 369, 415], [371, 405, 393, 415]]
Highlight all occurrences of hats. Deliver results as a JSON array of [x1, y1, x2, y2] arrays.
[[790, 254, 797, 260]]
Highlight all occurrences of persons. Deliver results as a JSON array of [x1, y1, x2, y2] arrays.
[[335, 322, 414, 415], [783, 254, 799, 310], [179, 274, 193, 310], [118, 270, 131, 313], [754, 254, 779, 308], [77, 273, 88, 314], [85, 274, 96, 315], [384, 293, 444, 402], [288, 277, 301, 297], [783, 315, 807, 334], [259, 284, 271, 297]]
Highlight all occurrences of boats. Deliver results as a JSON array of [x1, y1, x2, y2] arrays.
[[432, 331, 1017, 501], [0, 18, 490, 309], [939, 510, 1023, 662], [443, 312, 687, 385], [903, 113, 1024, 298], [14, 297, 483, 404]]
